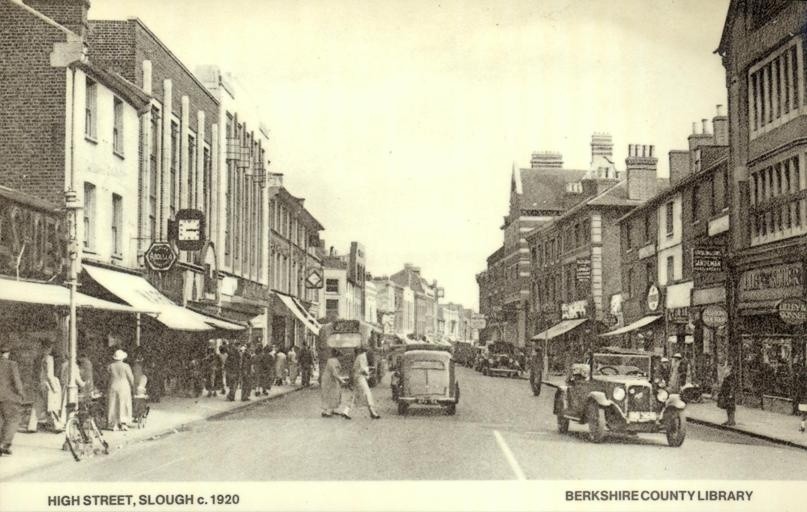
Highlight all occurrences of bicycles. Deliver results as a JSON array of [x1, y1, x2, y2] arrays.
[[65, 398, 109, 462]]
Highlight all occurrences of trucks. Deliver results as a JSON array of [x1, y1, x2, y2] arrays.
[[317, 318, 385, 389]]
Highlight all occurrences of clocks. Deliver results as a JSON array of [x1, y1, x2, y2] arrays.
[[168, 207, 206, 251]]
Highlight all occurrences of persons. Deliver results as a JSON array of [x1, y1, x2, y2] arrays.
[[657, 353, 738, 425], [0, 337, 149, 456], [320, 348, 349, 416], [183, 339, 315, 402], [529, 346, 544, 396], [341, 348, 381, 420]]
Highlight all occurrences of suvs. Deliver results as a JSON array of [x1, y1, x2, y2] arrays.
[[481, 342, 521, 377]]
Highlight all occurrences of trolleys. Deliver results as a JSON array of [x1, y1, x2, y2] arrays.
[[676, 373, 703, 403]]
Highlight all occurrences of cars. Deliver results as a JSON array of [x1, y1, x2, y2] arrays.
[[394, 349, 459, 415], [553, 354, 688, 447]]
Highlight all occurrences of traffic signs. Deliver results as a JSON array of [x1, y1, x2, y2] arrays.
[[693, 258, 723, 273], [693, 247, 724, 260]]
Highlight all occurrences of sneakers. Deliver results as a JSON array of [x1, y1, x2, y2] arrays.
[[321, 410, 353, 419], [207, 389, 268, 402], [110, 424, 129, 432], [275, 380, 314, 386], [0, 444, 13, 456], [722, 419, 737, 426], [26, 428, 66, 434], [370, 414, 381, 419]]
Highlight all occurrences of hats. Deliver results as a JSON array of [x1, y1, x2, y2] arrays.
[[673, 352, 682, 359], [660, 358, 670, 363], [112, 349, 128, 361]]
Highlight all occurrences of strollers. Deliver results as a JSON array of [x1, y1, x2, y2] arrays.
[[130, 383, 150, 429]]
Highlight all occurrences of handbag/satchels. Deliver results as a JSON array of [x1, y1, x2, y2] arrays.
[[716, 395, 726, 409]]
